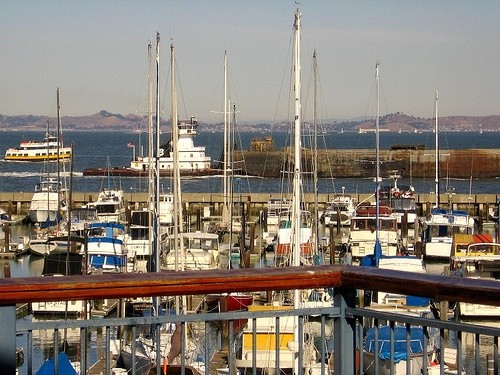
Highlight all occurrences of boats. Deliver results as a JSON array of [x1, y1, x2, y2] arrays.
[[0, 10, 499, 374]]
